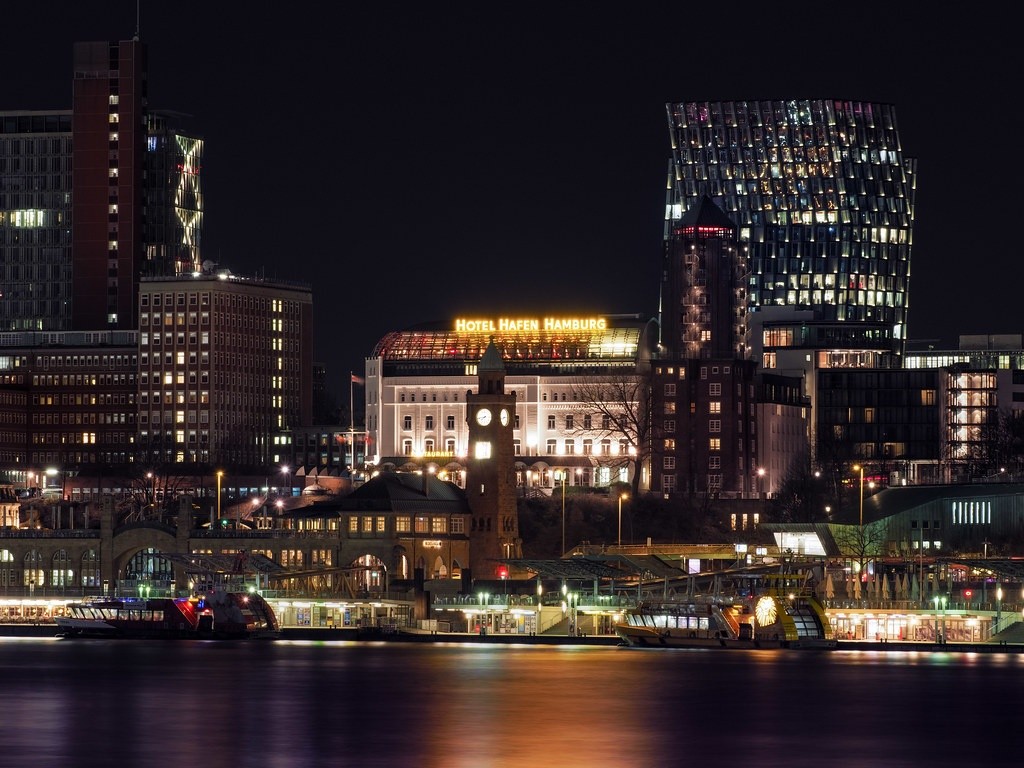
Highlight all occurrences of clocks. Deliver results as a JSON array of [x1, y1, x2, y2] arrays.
[[475, 407, 493, 427], [499, 409, 510, 427]]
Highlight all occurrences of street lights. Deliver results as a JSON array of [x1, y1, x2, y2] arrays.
[[853, 465, 864, 526], [758, 468, 764, 499], [825, 505, 830, 517], [216, 470, 224, 520], [618, 493, 628, 547]]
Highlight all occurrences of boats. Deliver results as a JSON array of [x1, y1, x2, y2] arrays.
[[611, 572, 836, 649], [55, 583, 280, 642]]
